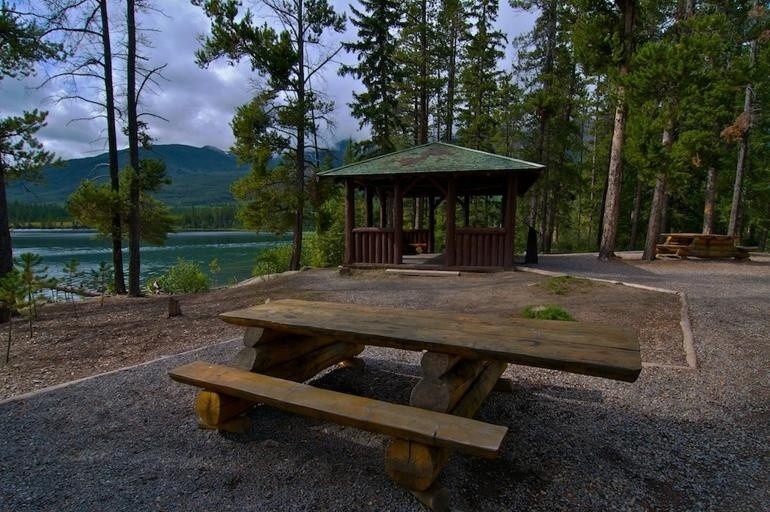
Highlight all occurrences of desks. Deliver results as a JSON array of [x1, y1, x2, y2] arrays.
[[219, 292, 641, 419], [659, 233, 740, 257]]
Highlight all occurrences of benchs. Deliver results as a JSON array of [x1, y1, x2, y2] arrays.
[[656, 243, 689, 259], [168, 361, 508, 491], [736, 246, 758, 262]]
